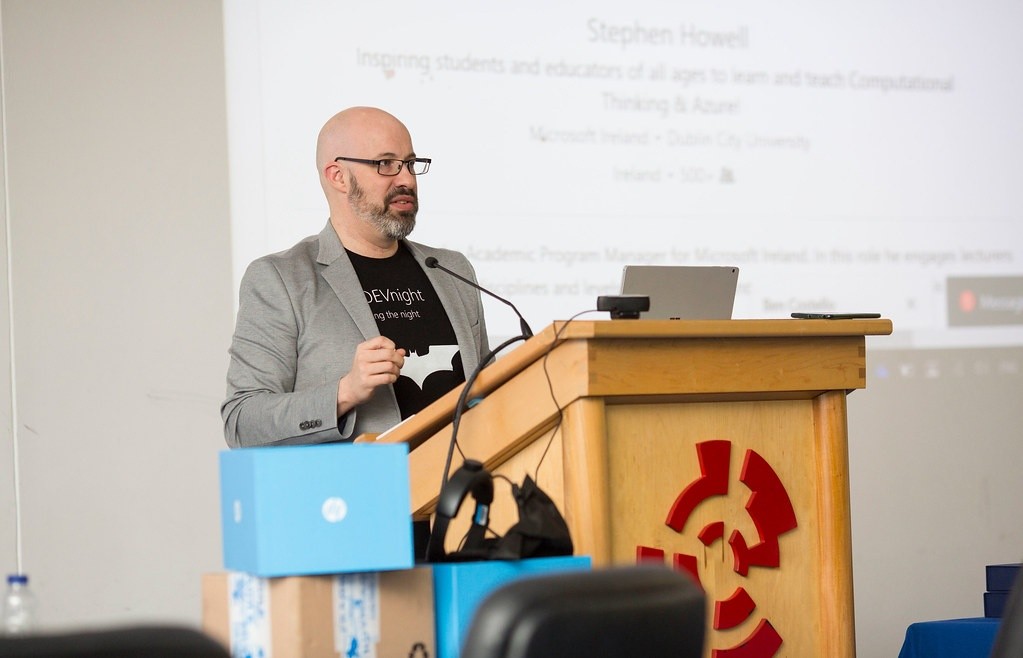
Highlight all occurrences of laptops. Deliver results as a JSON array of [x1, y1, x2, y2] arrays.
[[620, 266, 739, 319]]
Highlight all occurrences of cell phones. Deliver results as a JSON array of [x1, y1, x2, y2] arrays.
[[791, 312, 881, 319]]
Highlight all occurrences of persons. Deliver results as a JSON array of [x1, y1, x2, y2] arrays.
[[221, 106, 494, 449]]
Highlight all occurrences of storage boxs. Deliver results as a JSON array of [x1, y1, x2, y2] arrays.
[[197, 566, 434, 658], [218, 442, 415, 582], [414, 555, 592, 658]]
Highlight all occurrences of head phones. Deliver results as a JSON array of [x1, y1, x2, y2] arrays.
[[425, 458, 495, 563]]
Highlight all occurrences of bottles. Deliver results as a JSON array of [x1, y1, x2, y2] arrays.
[[0, 574, 38, 637]]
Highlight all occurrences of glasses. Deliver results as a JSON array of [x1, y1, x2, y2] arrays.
[[334, 157, 431, 176]]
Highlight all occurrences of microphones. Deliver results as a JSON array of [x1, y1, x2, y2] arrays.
[[424, 257, 534, 342]]
